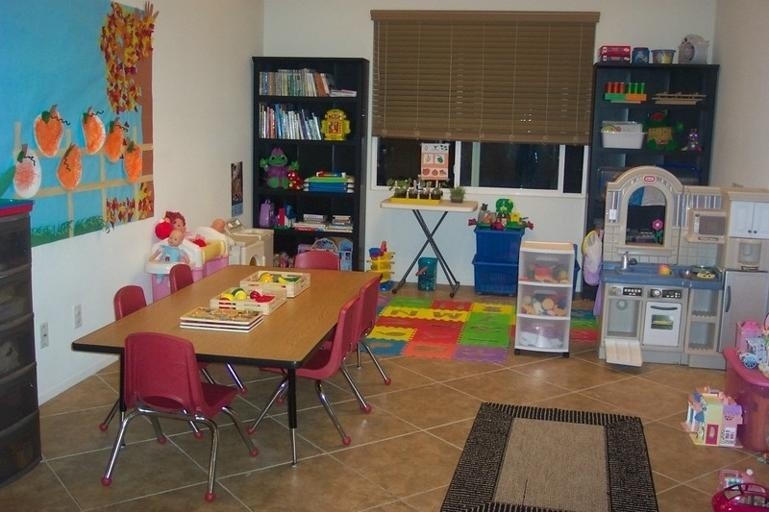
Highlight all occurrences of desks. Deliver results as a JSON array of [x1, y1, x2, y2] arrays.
[[71, 264, 384, 466], [380, 196, 478, 297]]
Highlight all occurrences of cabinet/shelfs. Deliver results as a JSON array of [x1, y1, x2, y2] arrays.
[[513, 240, 575, 357], [717, 269, 768, 354], [252, 56, 369, 274], [580, 61, 719, 300], [0, 199, 43, 489]]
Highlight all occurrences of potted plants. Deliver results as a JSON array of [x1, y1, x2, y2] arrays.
[[409, 189, 418, 198], [450, 187, 465, 202], [420, 189, 430, 199], [431, 187, 441, 200], [385, 178, 409, 198]]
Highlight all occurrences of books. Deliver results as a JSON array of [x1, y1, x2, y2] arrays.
[[259, 66, 356, 141], [290, 211, 354, 235]]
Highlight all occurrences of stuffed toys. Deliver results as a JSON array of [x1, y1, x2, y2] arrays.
[[258, 147, 300, 191]]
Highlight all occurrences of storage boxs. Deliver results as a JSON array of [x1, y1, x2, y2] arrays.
[[640, 167, 703, 206], [593, 166, 643, 207], [598, 44, 630, 64], [597, 125, 647, 148], [474, 223, 525, 264], [472, 253, 518, 297], [722, 348, 769, 453]]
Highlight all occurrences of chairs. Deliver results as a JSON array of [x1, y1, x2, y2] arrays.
[[319, 276, 391, 409], [99, 284, 214, 438], [101, 331, 259, 502], [248, 295, 371, 446], [287, 250, 340, 273], [169, 264, 248, 394]]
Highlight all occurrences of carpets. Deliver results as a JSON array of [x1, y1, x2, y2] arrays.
[[440, 401, 660, 512]]
